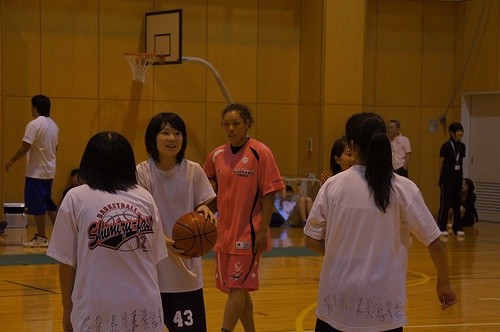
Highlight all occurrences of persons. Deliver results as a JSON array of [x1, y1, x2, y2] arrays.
[[5, 94, 81, 247], [202, 103, 284, 332], [133, 112, 219, 332], [46, 131, 170, 332], [302, 112, 457, 332], [276, 121, 480, 236]]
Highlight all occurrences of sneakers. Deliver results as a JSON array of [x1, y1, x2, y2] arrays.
[[23, 233, 49, 246]]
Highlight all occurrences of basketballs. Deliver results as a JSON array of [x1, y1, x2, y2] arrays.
[[172, 212, 217, 257]]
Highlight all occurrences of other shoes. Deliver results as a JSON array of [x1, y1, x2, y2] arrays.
[[440, 231, 448, 236], [457, 231, 464, 236]]
